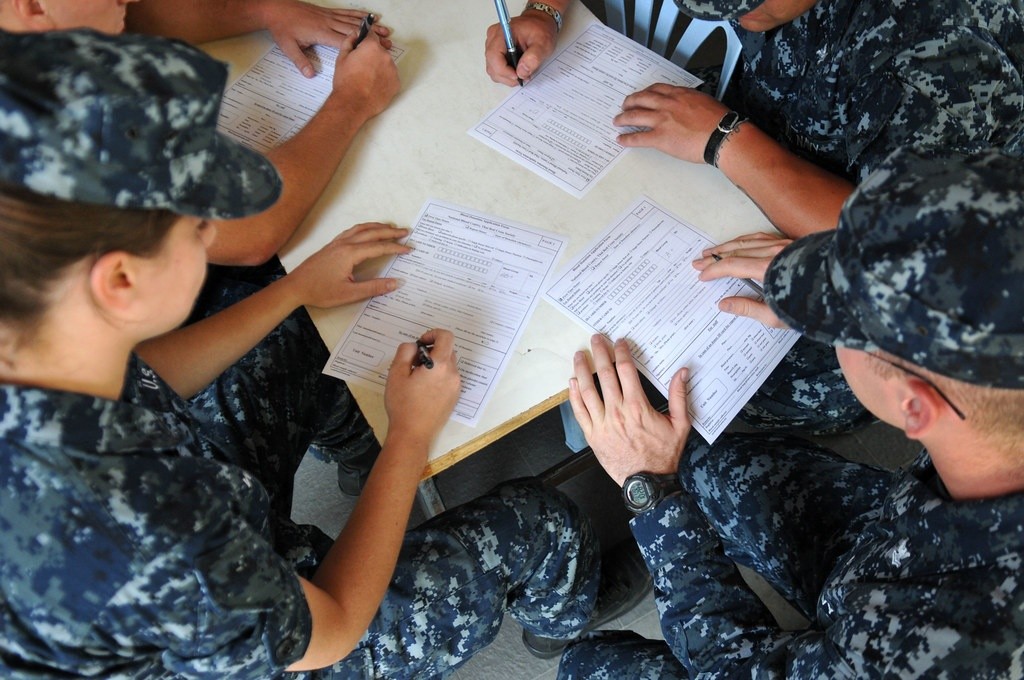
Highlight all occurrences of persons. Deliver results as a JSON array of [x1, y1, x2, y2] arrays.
[[0, 0, 403, 328], [559, 151, 1024, 680], [2, 24, 656, 676]]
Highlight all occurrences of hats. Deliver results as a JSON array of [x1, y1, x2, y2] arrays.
[[762, 151, 1024, 390], [4, 24, 282, 220]]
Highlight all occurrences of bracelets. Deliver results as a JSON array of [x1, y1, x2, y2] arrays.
[[524, 1, 563, 36], [484, 0, 1024, 243], [702, 108, 750, 167]]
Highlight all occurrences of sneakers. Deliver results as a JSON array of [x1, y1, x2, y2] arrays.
[[519, 536, 655, 660], [338, 457, 372, 498]]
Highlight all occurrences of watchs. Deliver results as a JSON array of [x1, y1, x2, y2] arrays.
[[619, 471, 682, 515]]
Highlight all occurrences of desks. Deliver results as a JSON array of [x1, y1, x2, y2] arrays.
[[189, 1, 790, 484]]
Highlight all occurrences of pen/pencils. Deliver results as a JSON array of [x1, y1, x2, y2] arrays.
[[357, 12, 375, 43], [709, 253, 767, 301], [415, 339, 434, 370], [494, 0, 526, 89]]
